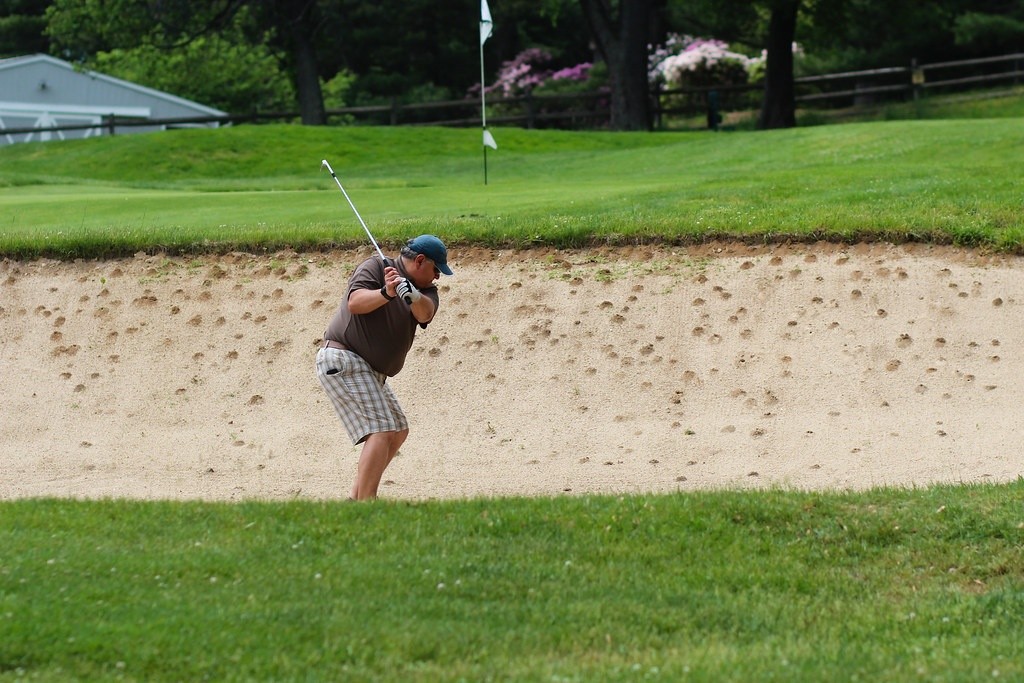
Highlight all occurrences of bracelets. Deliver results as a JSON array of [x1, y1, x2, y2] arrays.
[[381, 285, 396, 302]]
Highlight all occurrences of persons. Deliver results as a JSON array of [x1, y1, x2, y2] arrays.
[[316, 237, 454, 501]]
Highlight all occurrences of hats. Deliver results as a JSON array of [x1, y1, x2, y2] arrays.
[[410, 233, 452, 275]]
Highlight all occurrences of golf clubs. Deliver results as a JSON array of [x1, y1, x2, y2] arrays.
[[319, 158, 414, 307]]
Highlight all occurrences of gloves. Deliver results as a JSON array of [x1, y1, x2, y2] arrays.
[[393, 275, 422, 304]]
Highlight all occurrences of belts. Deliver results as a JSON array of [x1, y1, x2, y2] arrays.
[[320, 340, 345, 351]]
